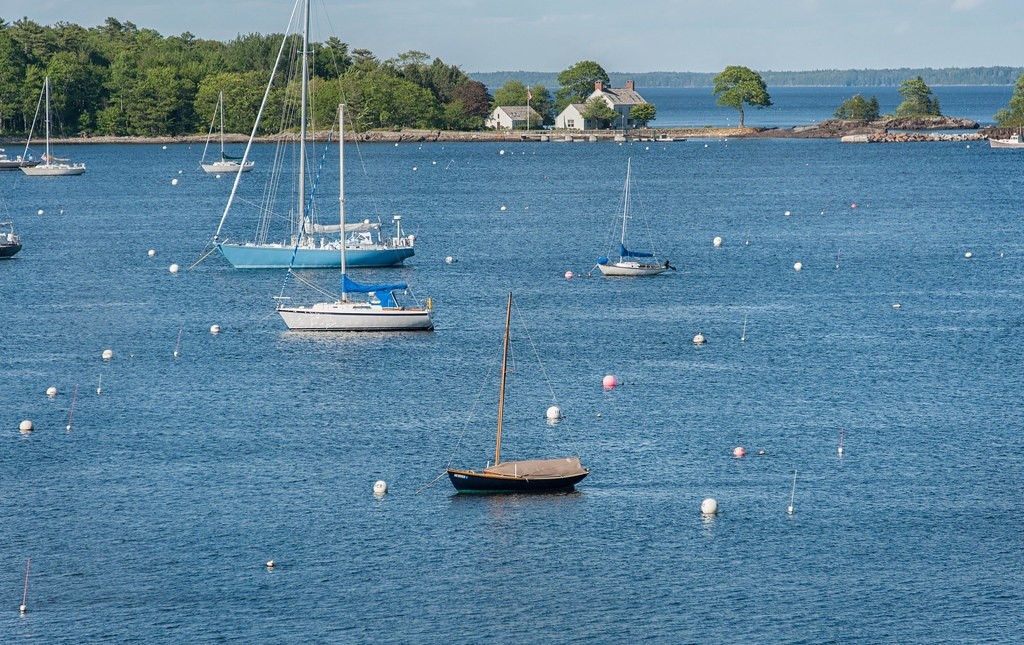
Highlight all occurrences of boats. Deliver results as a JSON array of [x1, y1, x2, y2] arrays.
[[988, 133, 1024, 148], [0, 221, 24, 260], [0, 152, 41, 168]]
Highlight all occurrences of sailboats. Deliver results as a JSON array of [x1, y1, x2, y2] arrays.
[[274, 105, 436, 331], [18, 75, 86, 176], [198, 90, 255, 173], [210, 2, 417, 271], [446, 291, 592, 495], [595, 155, 670, 276]]
